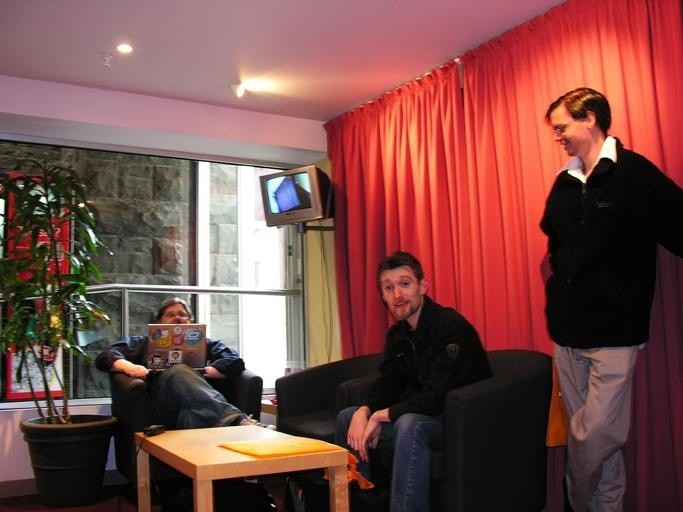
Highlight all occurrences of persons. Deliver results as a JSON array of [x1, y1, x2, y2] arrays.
[[94, 297, 277, 511], [539, 87, 683, 512], [335, 251, 493, 512]]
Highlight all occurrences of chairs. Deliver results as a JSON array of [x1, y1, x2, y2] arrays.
[[349, 350, 552, 512], [276, 352, 382, 512], [110, 370, 262, 501]]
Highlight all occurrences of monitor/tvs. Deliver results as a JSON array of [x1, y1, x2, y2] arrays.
[[259, 164, 334, 227]]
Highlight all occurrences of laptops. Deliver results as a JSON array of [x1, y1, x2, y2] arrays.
[[145, 323, 206, 395]]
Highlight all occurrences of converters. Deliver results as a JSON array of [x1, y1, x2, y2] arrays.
[[144, 424, 165, 436]]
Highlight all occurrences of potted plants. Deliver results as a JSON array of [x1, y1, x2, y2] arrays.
[[0, 152, 118, 507]]
